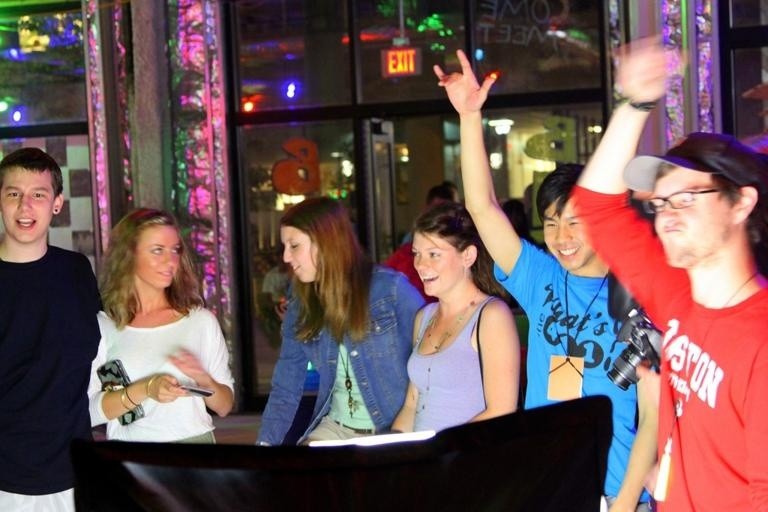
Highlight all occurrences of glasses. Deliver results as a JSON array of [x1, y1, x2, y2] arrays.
[[643, 184, 719, 215]]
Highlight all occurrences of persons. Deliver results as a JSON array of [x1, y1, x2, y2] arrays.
[[401, 177, 558, 239], [258, 244, 293, 349], [391, 204, 520, 432], [0, 148, 100, 511], [254, 199, 426, 444], [570, 31, 768, 509], [89, 206, 235, 445], [435, 48, 663, 512]]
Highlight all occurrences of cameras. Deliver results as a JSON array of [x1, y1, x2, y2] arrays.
[[607, 326, 663, 391]]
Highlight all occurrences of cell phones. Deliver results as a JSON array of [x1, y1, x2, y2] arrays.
[[178, 384, 214, 399]]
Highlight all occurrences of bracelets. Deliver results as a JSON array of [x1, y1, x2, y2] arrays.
[[124, 387, 139, 407], [120, 388, 132, 411], [144, 372, 156, 399], [615, 93, 656, 113]]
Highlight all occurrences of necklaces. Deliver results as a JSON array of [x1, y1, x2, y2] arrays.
[[340, 346, 355, 418], [406, 290, 482, 412], [675, 271, 760, 418]]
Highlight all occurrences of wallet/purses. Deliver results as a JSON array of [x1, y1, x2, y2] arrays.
[[96, 358, 145, 426]]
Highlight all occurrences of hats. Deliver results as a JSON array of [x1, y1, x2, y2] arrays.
[[621, 132, 766, 193]]
